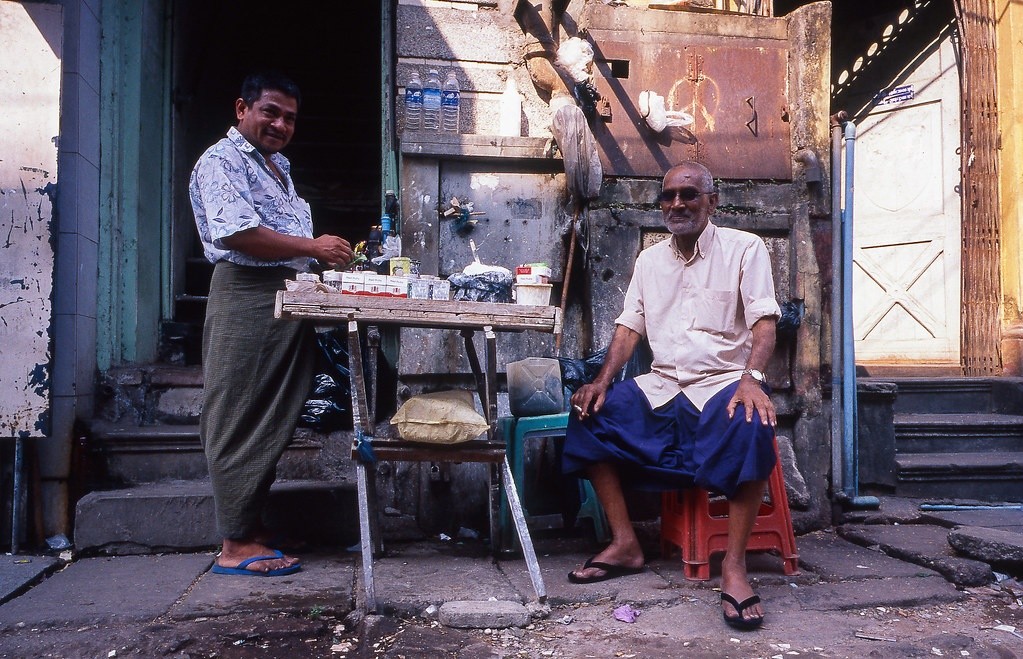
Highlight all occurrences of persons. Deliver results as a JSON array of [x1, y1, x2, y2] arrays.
[[559, 160, 778, 631], [187, 69, 354, 579]]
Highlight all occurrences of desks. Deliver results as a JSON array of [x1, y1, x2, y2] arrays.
[[273, 290, 564, 616]]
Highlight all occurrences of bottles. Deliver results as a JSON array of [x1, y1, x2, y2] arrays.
[[404, 70, 423, 129], [441, 72, 461, 133], [423, 70, 441, 130]]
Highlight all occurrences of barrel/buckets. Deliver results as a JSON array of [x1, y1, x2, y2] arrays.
[[514, 283, 553, 306]]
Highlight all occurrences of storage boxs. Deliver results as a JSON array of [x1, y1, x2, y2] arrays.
[[322, 269, 451, 301], [516, 266, 552, 284], [514, 282, 553, 306]]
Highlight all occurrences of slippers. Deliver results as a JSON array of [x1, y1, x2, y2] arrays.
[[568, 556, 644, 583], [721, 591, 764, 627], [212, 550, 301, 576]]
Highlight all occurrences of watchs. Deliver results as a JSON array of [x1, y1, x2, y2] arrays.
[[742, 368, 768, 384]]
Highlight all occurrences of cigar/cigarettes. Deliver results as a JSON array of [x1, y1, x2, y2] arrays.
[[573, 403, 590, 418]]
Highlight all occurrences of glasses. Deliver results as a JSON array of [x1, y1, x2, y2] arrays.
[[658, 190, 711, 201]]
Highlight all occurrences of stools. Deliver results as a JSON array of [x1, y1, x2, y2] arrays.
[[660, 423, 802, 582], [497, 410, 608, 550]]
